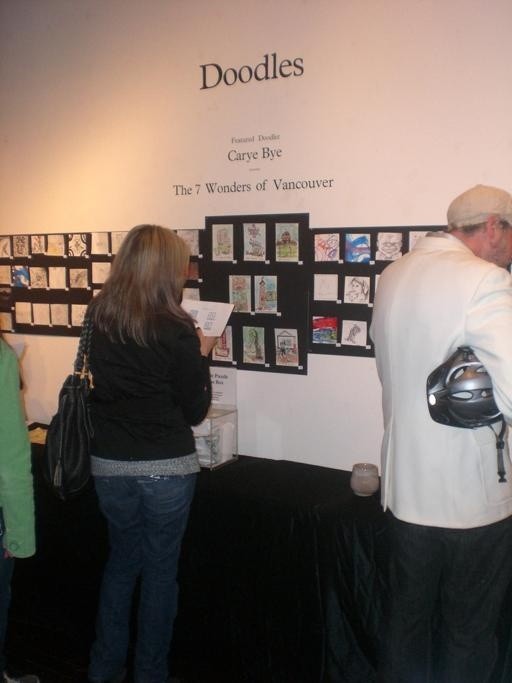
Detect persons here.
[84,224,217,683]
[368,183,512,681]
[0,325,35,629]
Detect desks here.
[27,423,385,683]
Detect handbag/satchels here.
[45,373,97,501]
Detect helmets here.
[426,347,503,427]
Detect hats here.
[446,184,512,230]
